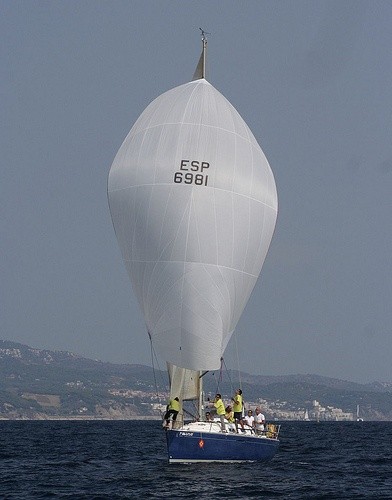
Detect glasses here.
[215,396,220,398]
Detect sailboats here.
[105,27,283,464]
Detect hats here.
[248,410,253,413]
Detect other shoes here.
[242,431,247,435]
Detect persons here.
[210,393,225,431]
[206,412,211,421]
[163,397,182,429]
[225,403,266,435]
[230,388,246,434]
